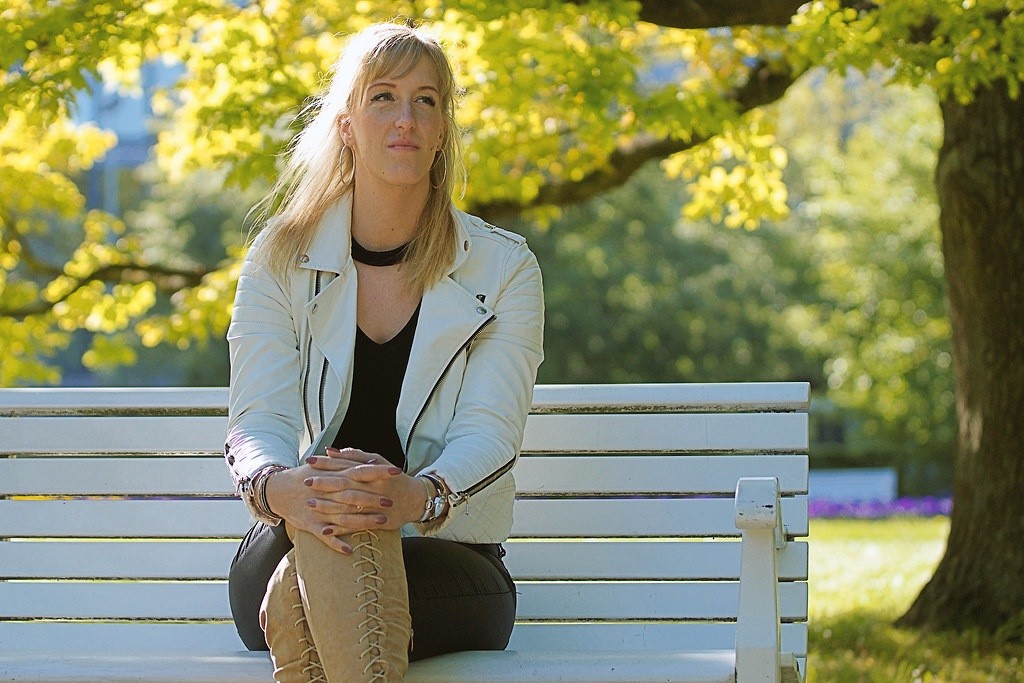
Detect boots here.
[259,546,326,680]
[283,516,412,683]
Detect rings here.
[356,505,363,512]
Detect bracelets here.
[253,467,286,520]
[419,477,433,523]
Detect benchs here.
[0,379,812,683]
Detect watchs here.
[418,473,447,523]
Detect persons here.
[223,21,545,683]
